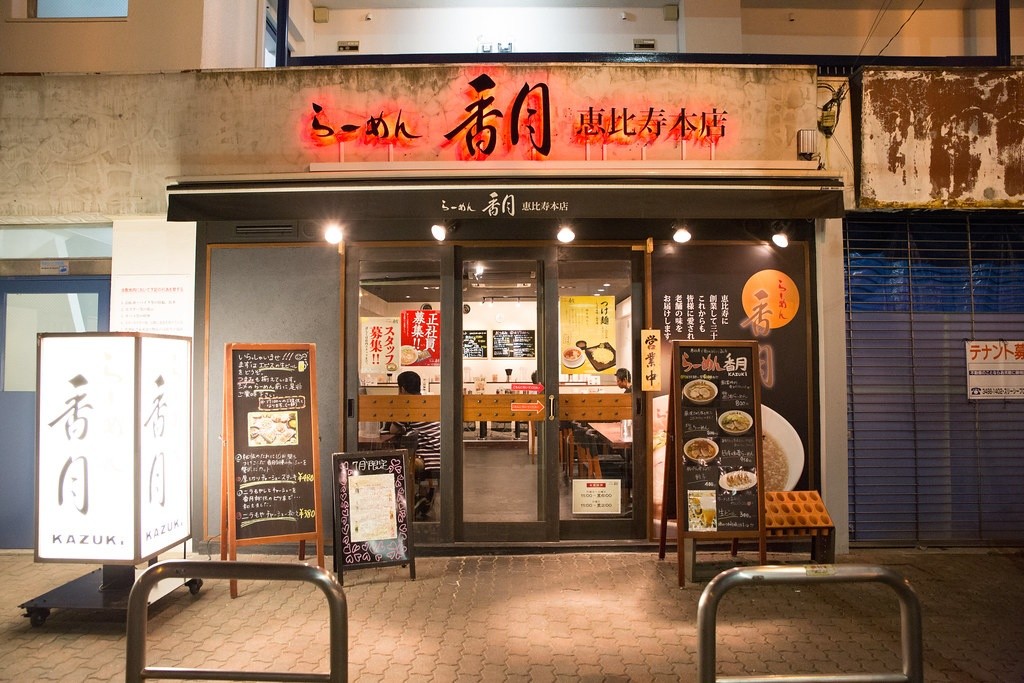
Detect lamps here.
[33,332,192,590]
[431,220,457,241]
[672,222,692,243]
[772,224,796,248]
[556,219,577,243]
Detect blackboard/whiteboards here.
[462,330,488,359]
[492,330,536,359]
[333,449,414,570]
[672,340,766,539]
[225,343,322,546]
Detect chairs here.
[531,421,626,479]
[400,431,419,521]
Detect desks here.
[587,423,632,449]
[359,433,398,449]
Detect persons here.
[614,368,632,393]
[387,370,441,519]
